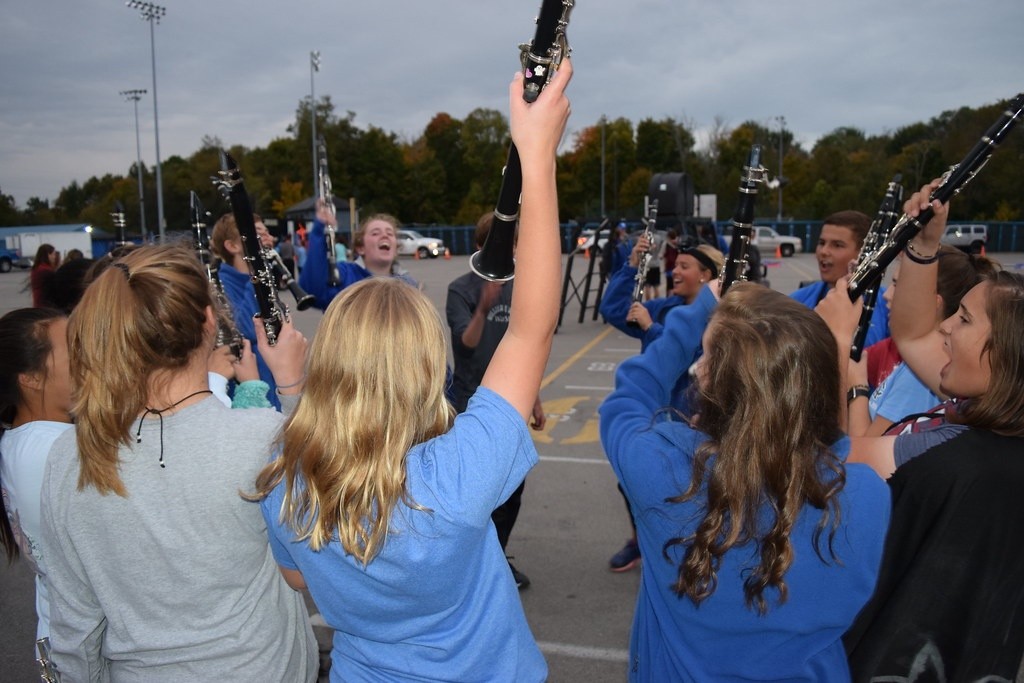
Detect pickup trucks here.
[723,226,803,259]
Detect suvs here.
[941,224,990,254]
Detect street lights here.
[118,87,151,243]
[773,114,788,222]
[309,49,323,203]
[599,114,612,221]
[980,245,986,256]
[123,1,168,245]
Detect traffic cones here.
[775,245,783,260]
[414,250,420,261]
[444,247,451,261]
[584,248,591,259]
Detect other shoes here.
[505,555,529,591]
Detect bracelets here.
[903,240,941,264]
[847,385,870,401]
[276,376,304,388]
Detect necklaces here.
[137,390,214,469]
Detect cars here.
[0,241,21,274]
[392,229,446,259]
[576,220,642,253]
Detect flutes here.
[468,0,583,282]
[626,180,666,322]
[320,137,345,282]
[850,173,906,364]
[845,93,1024,304]
[209,147,294,344]
[716,147,769,300]
[188,191,244,359]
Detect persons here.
[41,245,321,683]
[298,200,452,395]
[787,210,890,349]
[31,244,96,315]
[597,279,891,683]
[445,213,546,591]
[813,178,1024,683]
[598,238,724,571]
[212,212,282,414]
[864,338,903,388]
[86,245,271,409]
[608,228,729,300]
[237,56,575,683]
[0,309,238,683]
[276,234,347,279]
[847,243,1002,437]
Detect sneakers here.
[608,539,642,572]
